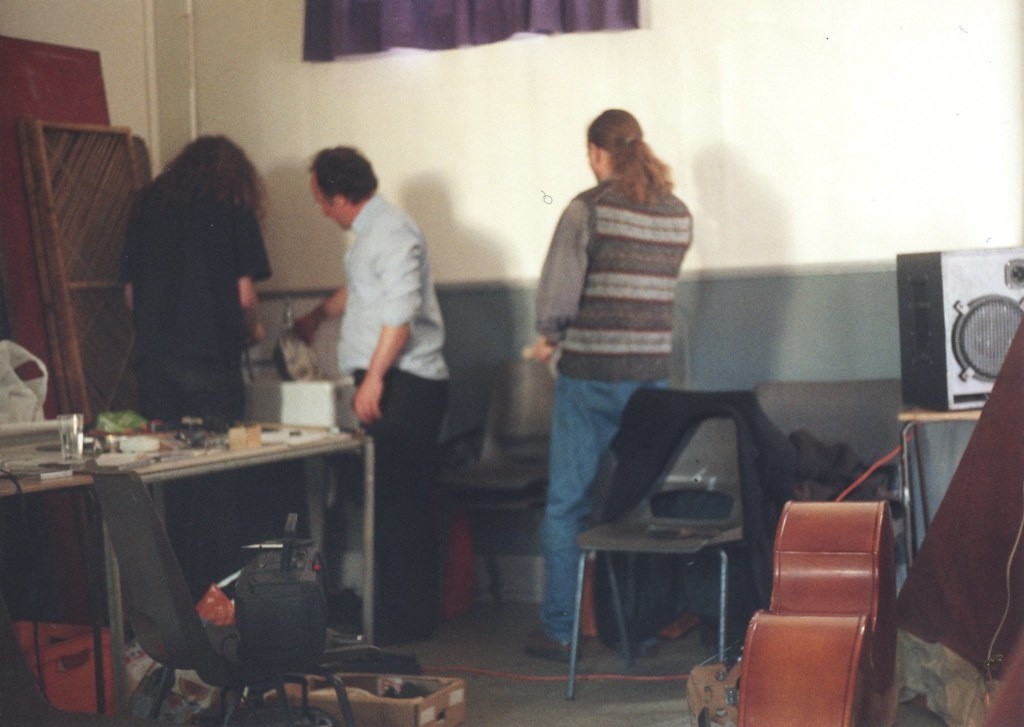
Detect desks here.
[898,407,983,576]
[0,426,375,727]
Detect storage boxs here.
[263,673,466,727]
[242,377,362,430]
[10,620,117,715]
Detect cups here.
[57,413,84,461]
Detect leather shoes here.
[522,635,584,662]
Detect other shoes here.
[358,612,443,644]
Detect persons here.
[121,134,272,602]
[293,145,450,648]
[529,108,692,661]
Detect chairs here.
[436,357,744,700]
[0,470,380,727]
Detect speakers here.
[897,248,1024,412]
